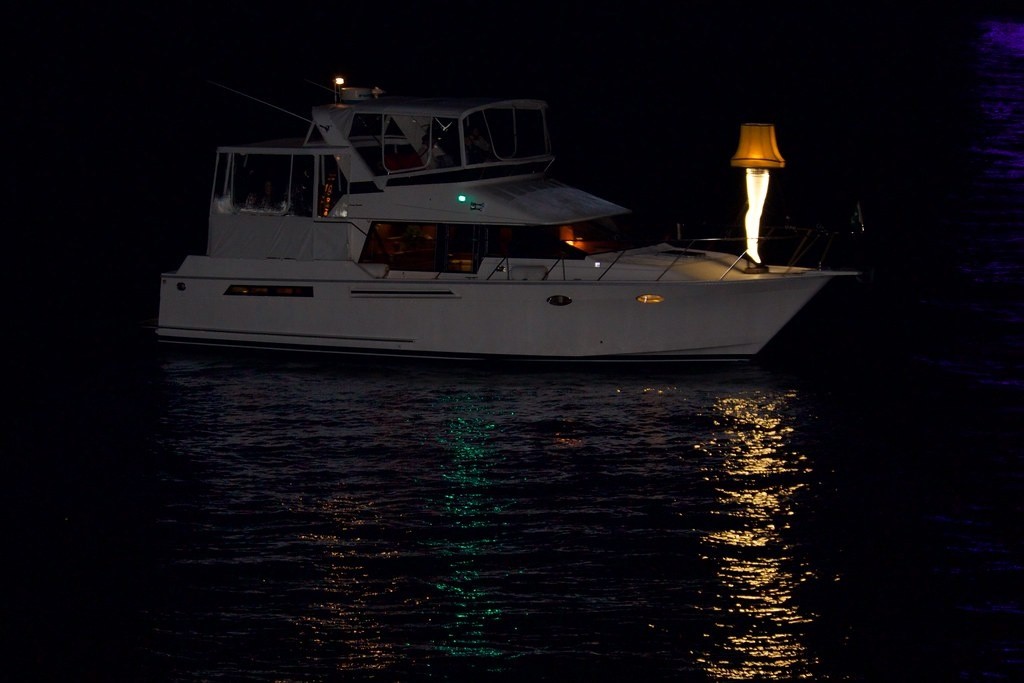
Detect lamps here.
[731,124,785,274]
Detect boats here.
[154,88,859,378]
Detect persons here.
[243,180,277,209]
[416,121,508,180]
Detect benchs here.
[382,152,426,175]
